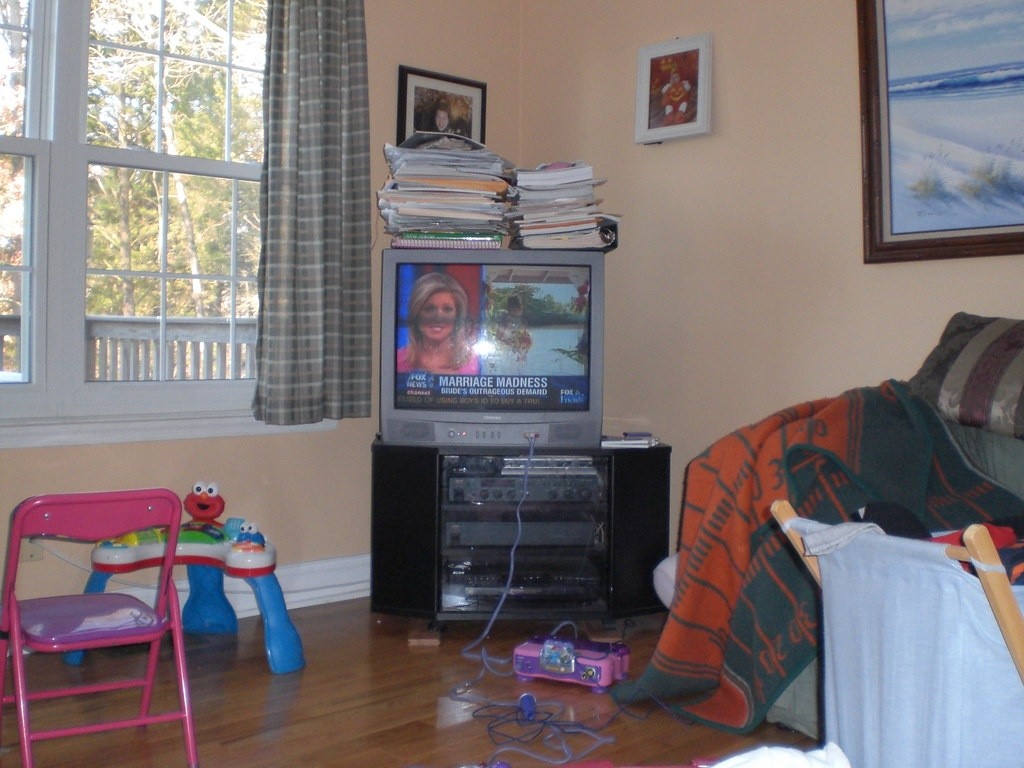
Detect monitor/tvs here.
[379,248,605,450]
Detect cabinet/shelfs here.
[368,444,673,632]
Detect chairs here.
[1,480,202,767]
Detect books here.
[377,130,617,253]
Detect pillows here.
[910,309,1024,442]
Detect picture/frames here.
[856,1,1024,264]
[395,63,488,150]
[635,31,712,143]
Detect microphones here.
[520,694,537,721]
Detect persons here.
[395,272,480,374]
[662,72,690,124]
[496,294,530,361]
[425,97,455,134]
